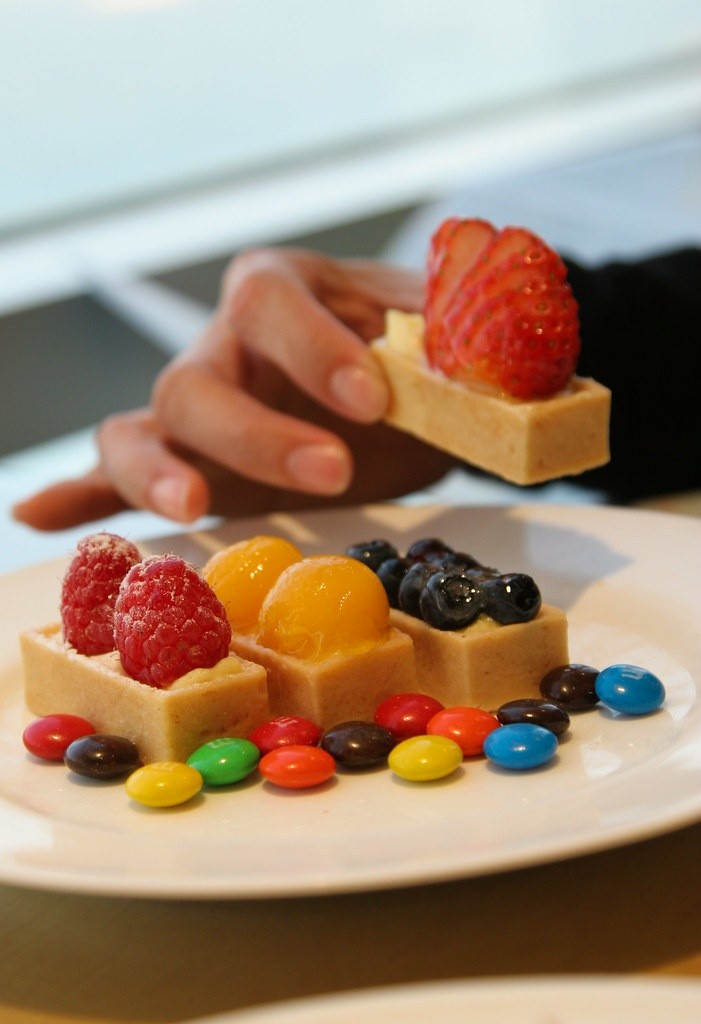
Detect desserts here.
[356,214,610,486]
[20,531,569,768]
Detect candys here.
[20,663,665,809]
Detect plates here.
[179,972,701,1024]
[0,504,701,899]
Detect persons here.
[8,243,701,532]
